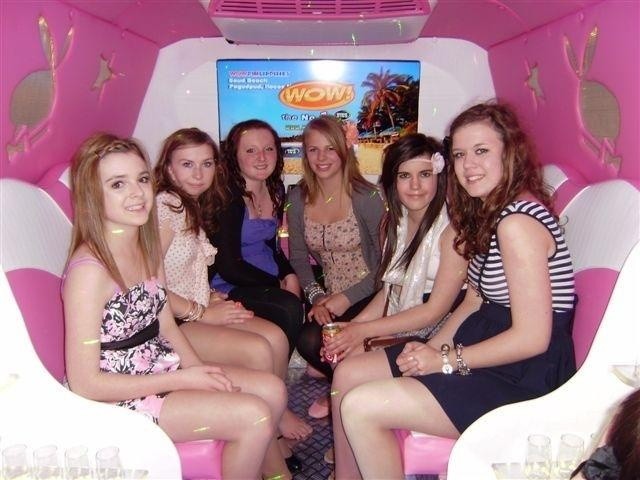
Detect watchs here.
[440,342,453,375]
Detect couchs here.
[1,157,257,479]
[374,160,640,474]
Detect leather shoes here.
[304,362,334,419]
[273,433,303,474]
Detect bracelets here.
[175,299,206,323]
[303,280,325,305]
[455,343,471,376]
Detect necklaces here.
[256,205,262,217]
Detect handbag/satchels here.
[361,283,426,348]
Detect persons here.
[331,102,579,480]
[285,114,387,420]
[207,118,313,441]
[319,133,455,480]
[154,127,313,480]
[59,130,288,480]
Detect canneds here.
[321,325,343,364]
